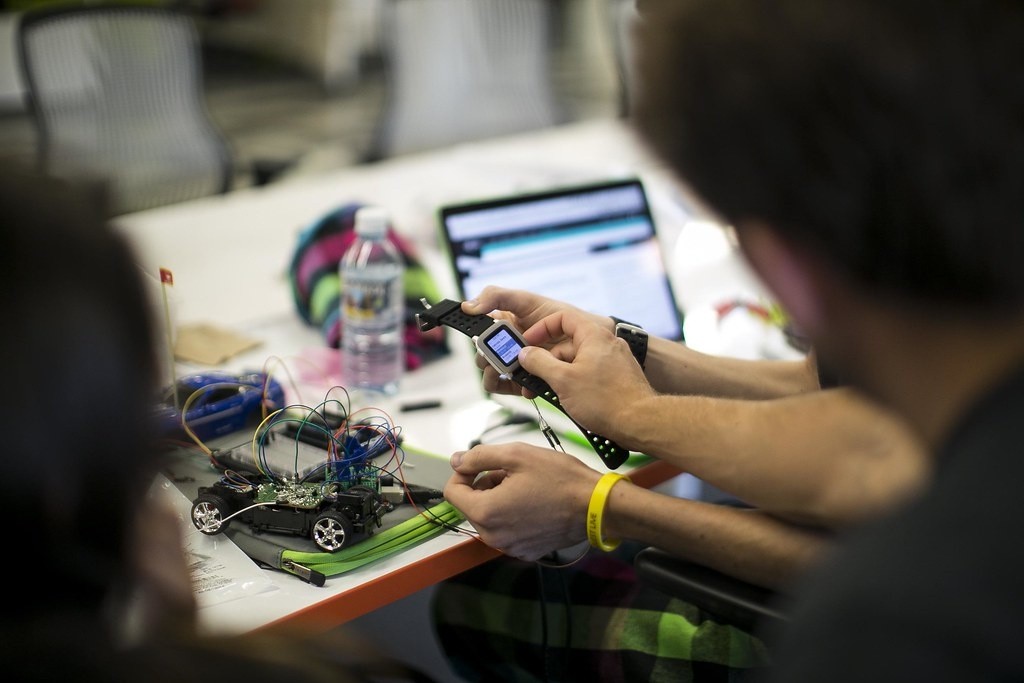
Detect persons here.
[444,0,1024,683]
[2,139,439,683]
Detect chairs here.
[14,0,643,218]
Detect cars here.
[155,372,285,449]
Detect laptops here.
[435,175,692,467]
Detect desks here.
[108,115,805,644]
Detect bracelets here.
[584,470,632,555]
[604,306,650,382]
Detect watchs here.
[402,290,630,470]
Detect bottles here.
[340,206,404,406]
